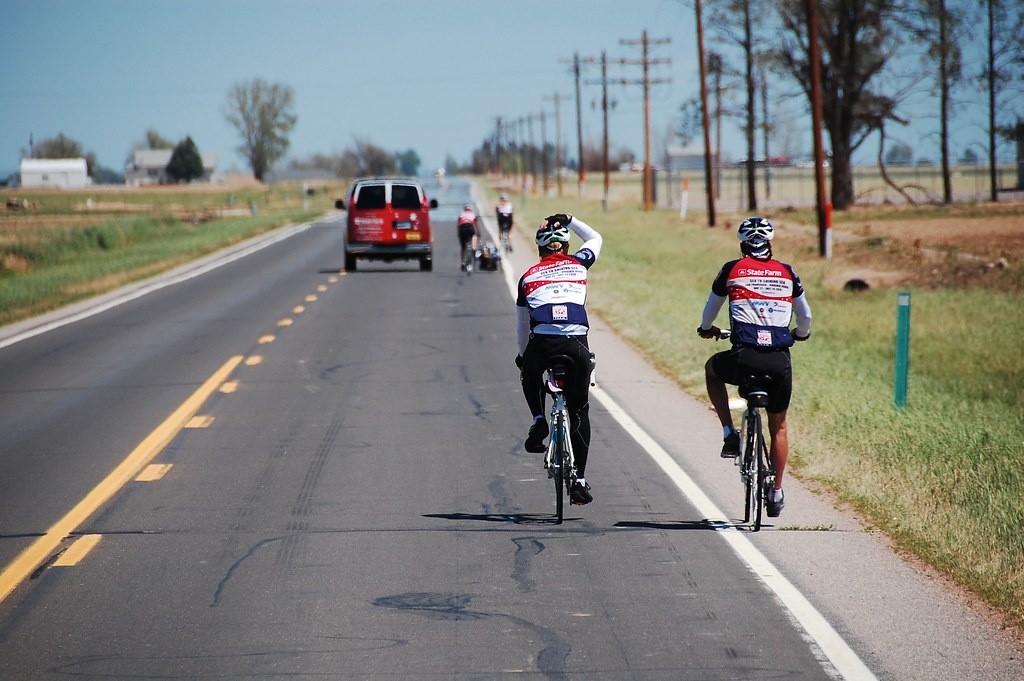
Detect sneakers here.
[721,433,741,457]
[570,481,593,505]
[766,488,784,517]
[524,417,550,452]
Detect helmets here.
[536,221,571,248]
[463,202,471,210]
[498,193,508,200]
[737,216,775,248]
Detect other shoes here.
[461,262,466,272]
[508,245,514,253]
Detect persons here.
[456,204,481,274]
[516,214,603,506]
[699,216,812,518]
[495,194,514,252]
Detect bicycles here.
[463,233,480,276]
[515,331,596,524]
[498,219,513,253]
[698,323,809,533]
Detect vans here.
[335,176,439,272]
